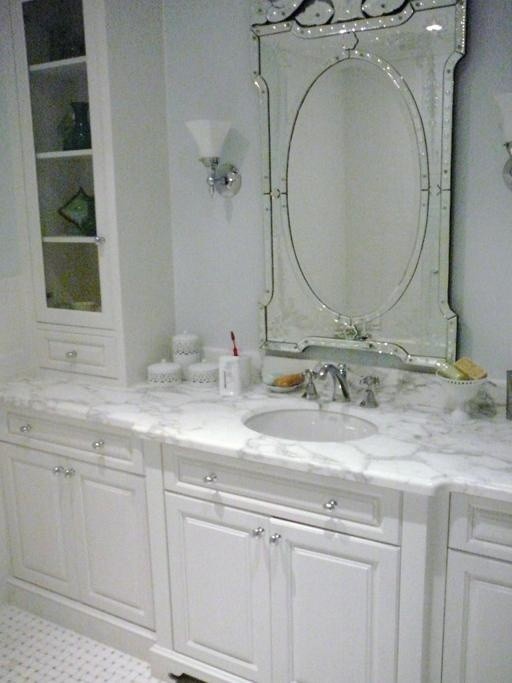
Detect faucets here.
[317,362,353,403]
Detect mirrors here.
[248,0,468,372]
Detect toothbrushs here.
[231,331,238,356]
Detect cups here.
[218,354,252,397]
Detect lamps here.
[489,91,512,189]
[183,115,241,199]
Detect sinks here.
[240,402,378,444]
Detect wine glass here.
[435,367,488,424]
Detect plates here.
[266,381,308,391]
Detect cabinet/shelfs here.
[10,1,177,384]
[439,491,511,683]
[164,449,426,682]
[0,411,151,628]
